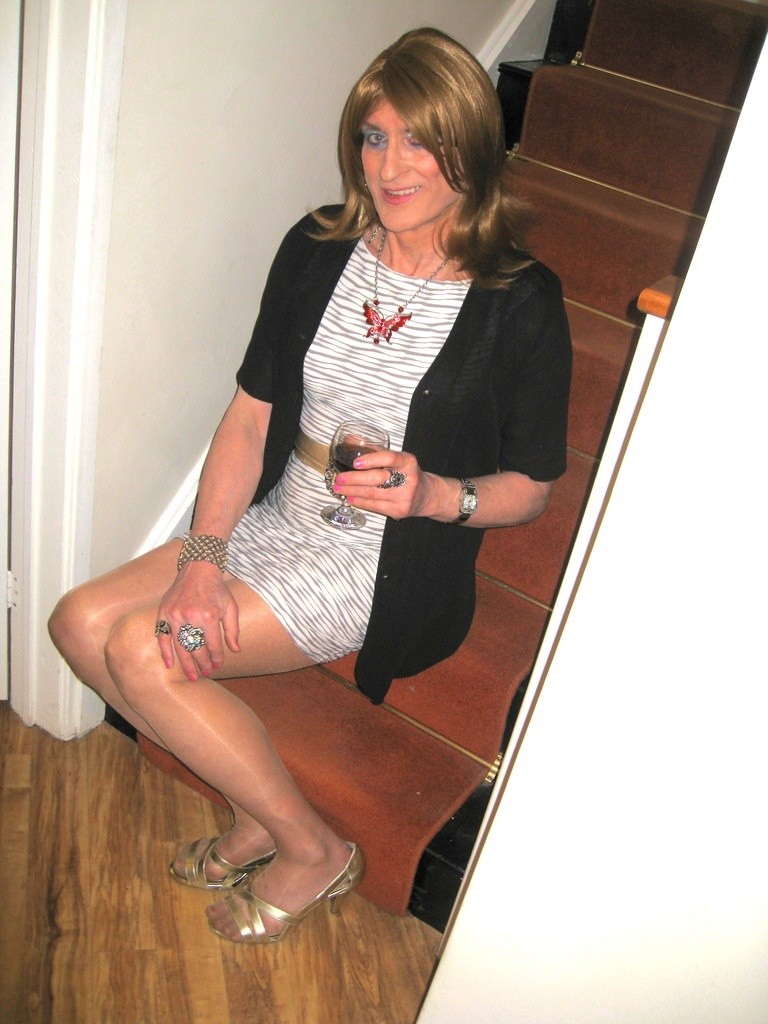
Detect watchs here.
[441,476,478,525]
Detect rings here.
[379,468,407,489]
[155,621,172,637]
[177,624,206,651]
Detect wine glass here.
[322,421,390,529]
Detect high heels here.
[168,833,278,892]
[207,841,366,944]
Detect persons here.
[48,29,575,944]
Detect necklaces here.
[362,230,447,345]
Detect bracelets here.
[178,534,229,575]
